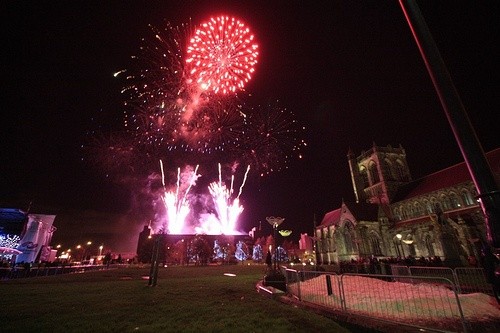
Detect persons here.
[335,254,447,275]
[266,249,272,273]
[2,256,92,274]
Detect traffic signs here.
[147,238,160,287]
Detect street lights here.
[265,217,284,276]
[279,230,291,266]
[396,233,406,260]
